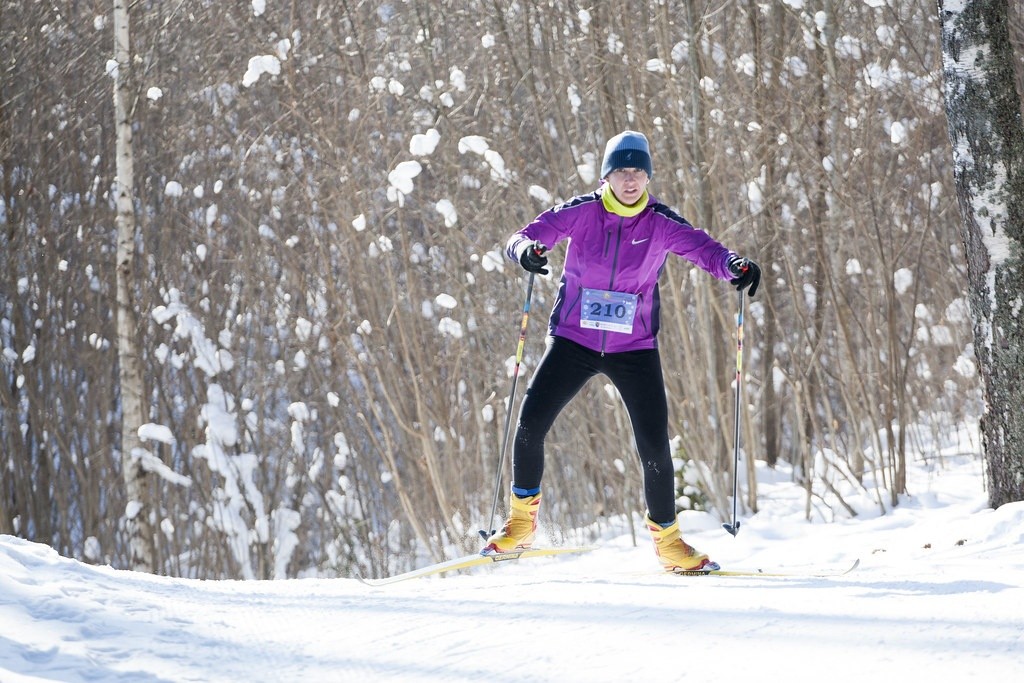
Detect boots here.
[646,509,709,573]
[488,490,542,555]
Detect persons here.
[486,130,762,570]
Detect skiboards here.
[478,528,721,579]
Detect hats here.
[601,130,653,180]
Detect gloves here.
[728,258,760,297]
[521,244,549,275]
